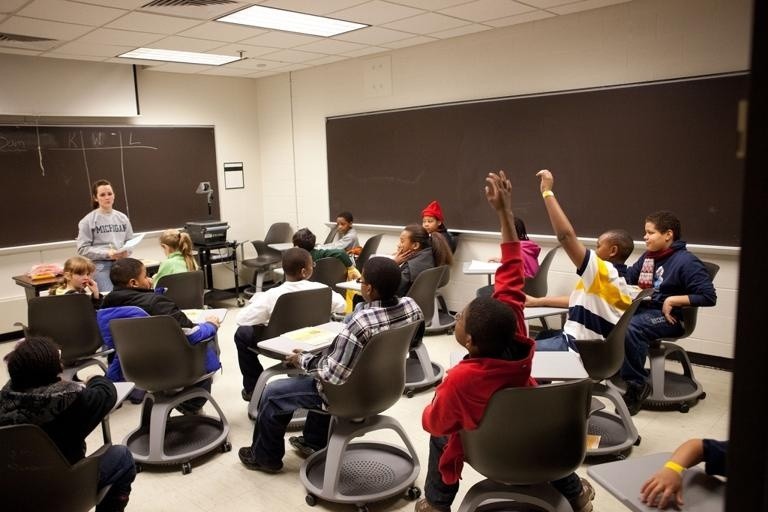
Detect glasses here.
[356,278,364,283]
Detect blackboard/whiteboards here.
[0,123,224,255]
[323,70,747,256]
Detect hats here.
[422,200,443,221]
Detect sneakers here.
[175,405,203,416]
[570,478,595,512]
[242,389,253,400]
[239,447,283,473]
[415,498,450,512]
[289,436,315,455]
[616,383,650,416]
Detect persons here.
[232,201,453,472]
[0,336,136,512]
[147,228,200,297]
[638,437,731,511]
[76,179,137,292]
[94,257,222,419]
[46,256,103,326]
[413,171,716,512]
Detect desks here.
[586,450,726,510]
